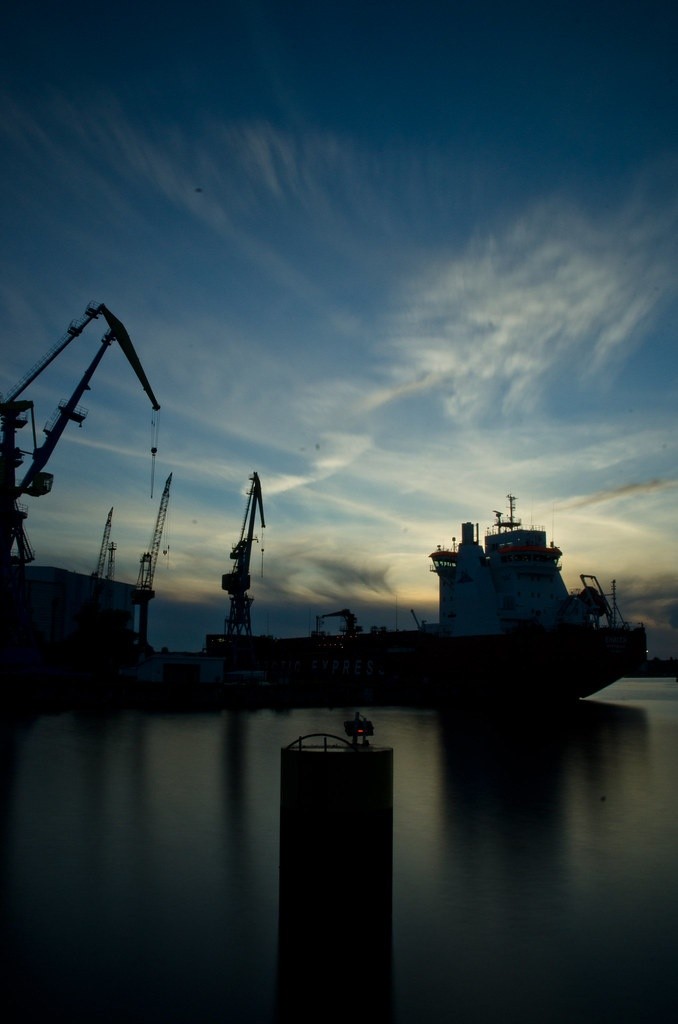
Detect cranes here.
[226,472,272,636]
[0,290,163,575]
[105,540,121,579]
[133,473,174,664]
[86,503,113,577]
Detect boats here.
[166,493,649,713]
